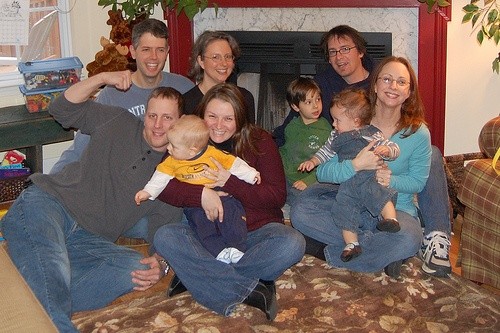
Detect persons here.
[272,25,454,279]
[0,70,184,333]
[150,84,306,322]
[183,30,256,126]
[298,86,401,262]
[48,18,196,241]
[291,56,431,278]
[135,115,262,264]
[278,77,336,205]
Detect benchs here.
[0,104,74,174]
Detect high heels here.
[244,280,275,322]
[168,272,187,297]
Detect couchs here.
[456,114,500,289]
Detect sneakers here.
[420,234,452,278]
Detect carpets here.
[71,254,500,333]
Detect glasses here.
[376,76,411,86]
[327,46,358,57]
[203,55,235,62]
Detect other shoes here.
[376,219,400,233]
[384,260,402,279]
[341,243,362,262]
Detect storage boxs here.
[18,56,84,89]
[19,85,66,114]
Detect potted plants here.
[99,0,218,57]
